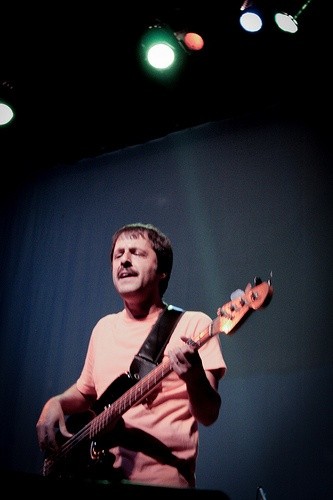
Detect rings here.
[175,358,179,363]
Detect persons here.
[35,222,227,488]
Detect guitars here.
[41,276,273,494]
